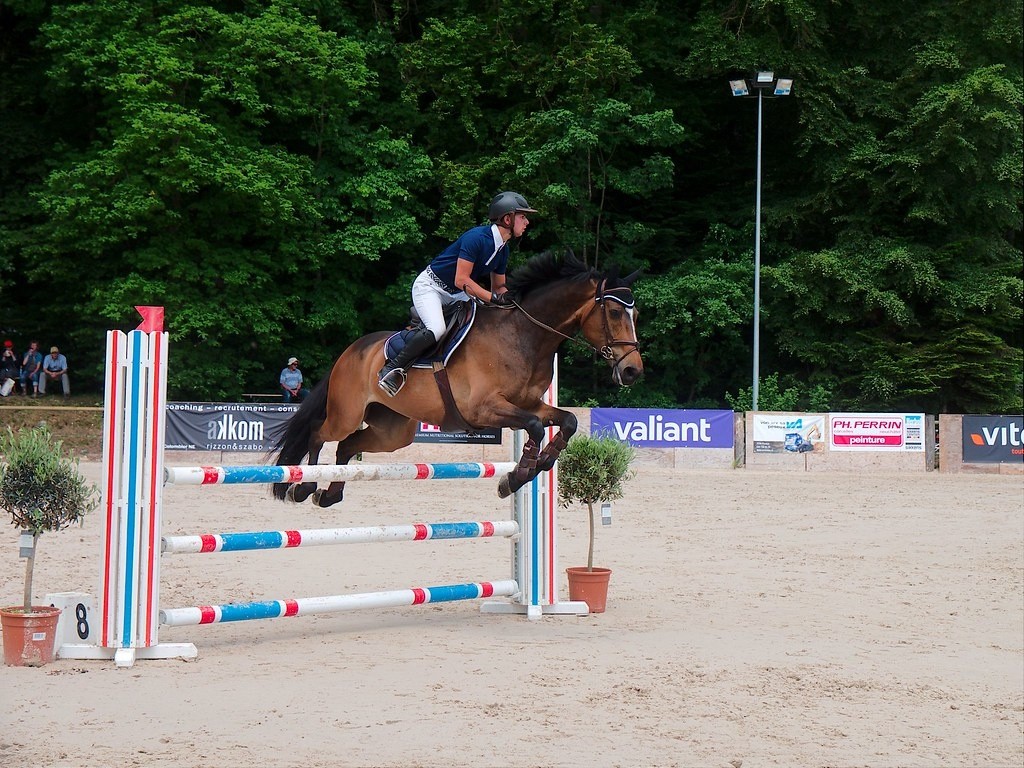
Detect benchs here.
[241,393,284,402]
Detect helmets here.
[489,192,538,228]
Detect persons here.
[19,342,42,398]
[376,191,538,392]
[280,358,310,403]
[0,338,18,396]
[38,347,71,396]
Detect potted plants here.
[0,424,102,667]
[558,420,637,614]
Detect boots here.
[376,328,436,393]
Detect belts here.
[426,265,454,294]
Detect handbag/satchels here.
[6,367,18,377]
[0,378,15,396]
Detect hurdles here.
[57,328,590,661]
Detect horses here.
[257,249,646,508]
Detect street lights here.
[730,68,795,411]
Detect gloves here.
[491,289,520,305]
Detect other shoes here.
[39,392,43,397]
[21,391,27,397]
[10,391,16,396]
[31,393,37,398]
[64,394,70,400]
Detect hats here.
[50,347,58,354]
[3,340,13,350]
[287,357,299,366]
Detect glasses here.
[293,364,298,366]
